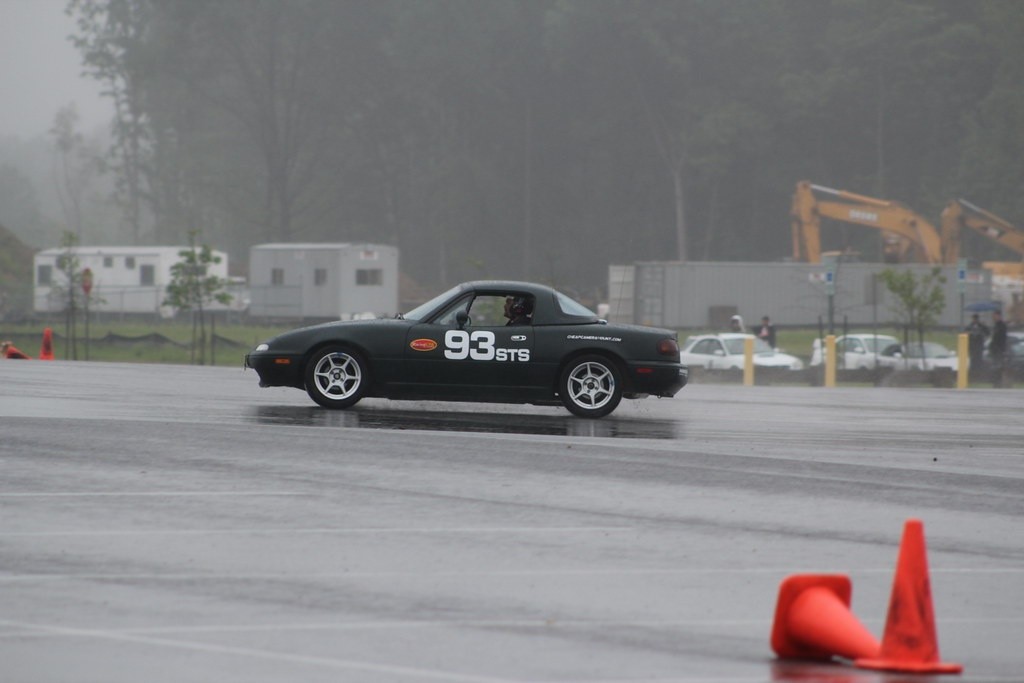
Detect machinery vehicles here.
[783,178,1022,277]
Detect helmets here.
[505,294,533,314]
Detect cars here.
[982,331,1024,367]
[809,333,906,380]
[242,279,688,421]
[873,340,962,388]
[679,331,805,382]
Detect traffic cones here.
[38,327,54,361]
[769,572,881,657]
[6,345,32,361]
[854,517,964,675]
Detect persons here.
[503,294,533,327]
[729,314,747,334]
[989,314,1007,387]
[964,315,989,385]
[757,315,777,350]
